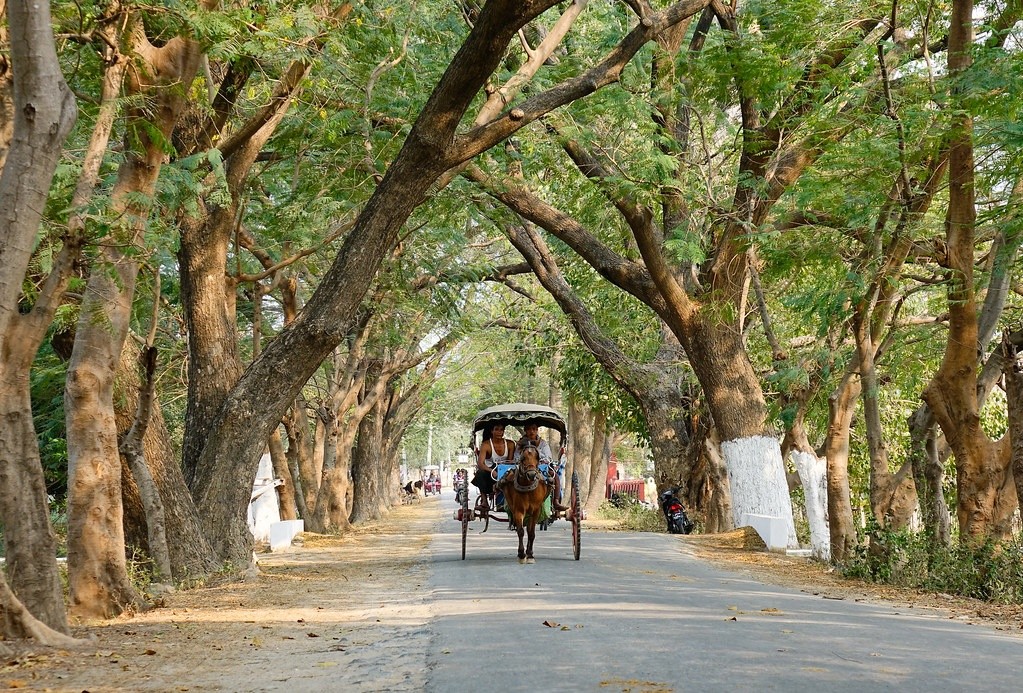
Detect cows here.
[403,480,423,505]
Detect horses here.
[503,437,548,564]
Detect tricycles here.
[421,464,442,497]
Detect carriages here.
[453,401,588,561]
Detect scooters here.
[454,480,469,506]
[656,484,694,535]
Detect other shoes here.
[475,504,490,512]
[553,505,569,511]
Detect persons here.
[405,466,468,496]
[512,422,569,511]
[471,421,516,510]
